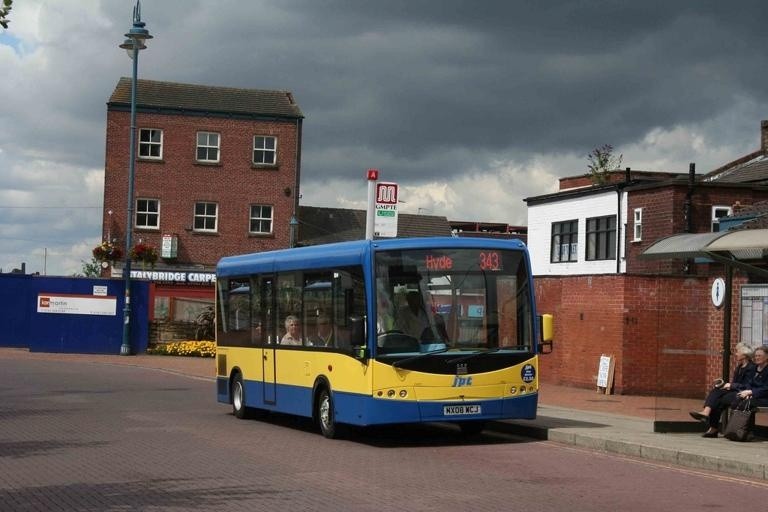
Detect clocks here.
[711,278,726,308]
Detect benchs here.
[722,407,767,437]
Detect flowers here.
[129,244,158,264]
[91,241,118,261]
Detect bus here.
[213,237,555,440]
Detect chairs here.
[348,317,367,347]
[235,307,250,330]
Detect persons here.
[689,341,757,438]
[374,298,396,334]
[279,313,313,345]
[730,346,767,442]
[305,313,344,349]
[394,290,454,345]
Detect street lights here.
[119,0,153,356]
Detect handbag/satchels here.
[723,397,752,441]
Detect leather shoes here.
[688,412,709,422]
[702,431,719,438]
